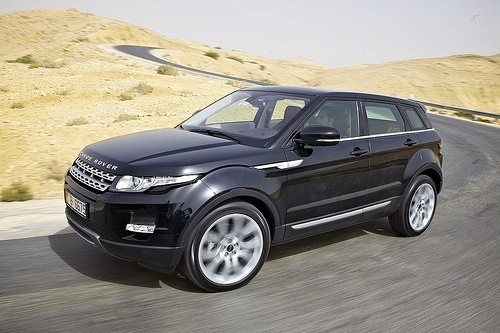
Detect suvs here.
[61,85,445,293]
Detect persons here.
[317,106,338,128]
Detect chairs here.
[270,106,302,132]
